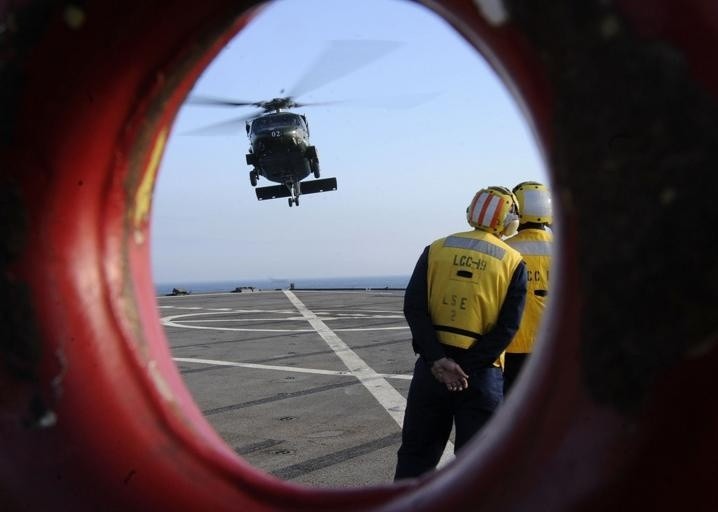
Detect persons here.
[502,180,556,400]
[388,183,527,482]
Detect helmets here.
[513,181,552,225]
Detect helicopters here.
[177,39,442,207]
